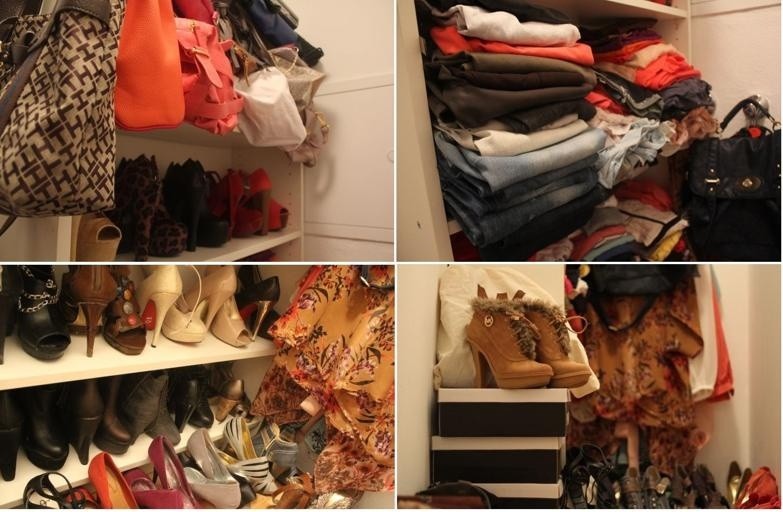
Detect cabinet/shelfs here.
[396,0,695,260]
[2,119,306,262]
[0,264,309,509]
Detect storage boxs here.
[431,435,568,498]
[436,385,573,437]
[467,478,562,510]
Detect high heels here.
[466,284,590,390]
[78,154,287,261]
[0,265,315,508]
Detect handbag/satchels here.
[0,0,329,217]
[686,97,781,262]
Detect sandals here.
[562,444,751,509]
